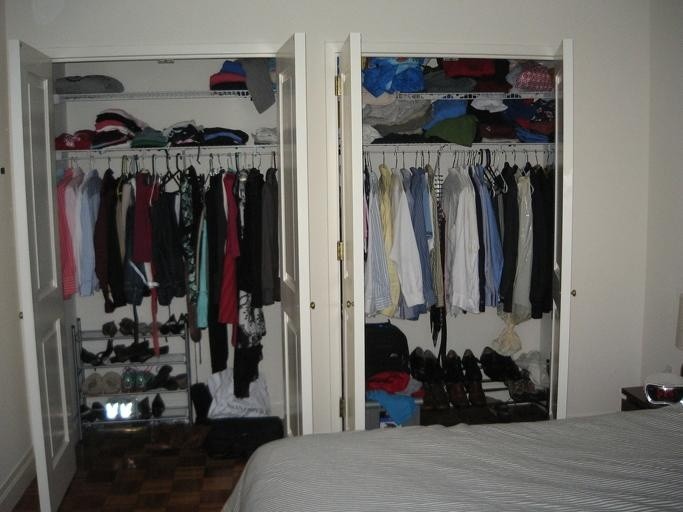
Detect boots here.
[171,383,212,450]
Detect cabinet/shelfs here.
[6,32,312,512]
[324,30,572,431]
[70,315,193,430]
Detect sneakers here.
[79,312,186,422]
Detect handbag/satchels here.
[207,417,284,459]
[366,324,409,371]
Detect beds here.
[222,401,683,512]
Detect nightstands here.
[620,386,683,412]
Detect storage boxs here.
[365,398,423,431]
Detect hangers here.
[365,143,553,171]
[67,144,278,192]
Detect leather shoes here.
[411,347,550,411]
